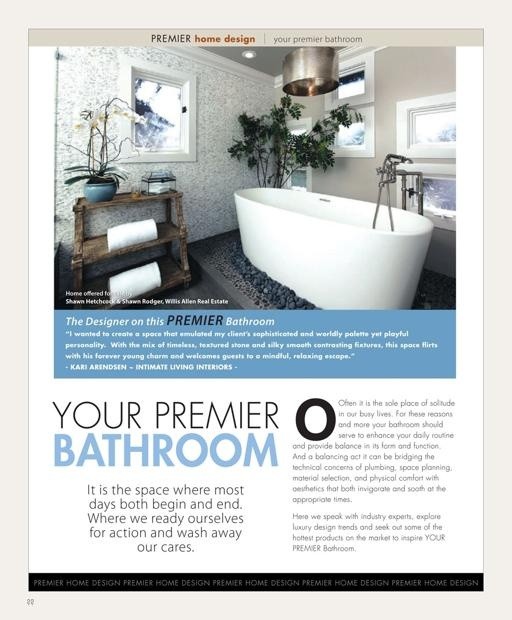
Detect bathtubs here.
[231,187,435,310]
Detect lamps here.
[281,48,341,98]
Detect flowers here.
[63,96,148,188]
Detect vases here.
[83,183,116,203]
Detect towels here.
[108,261,162,307]
[106,218,159,252]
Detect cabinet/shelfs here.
[70,191,192,310]
